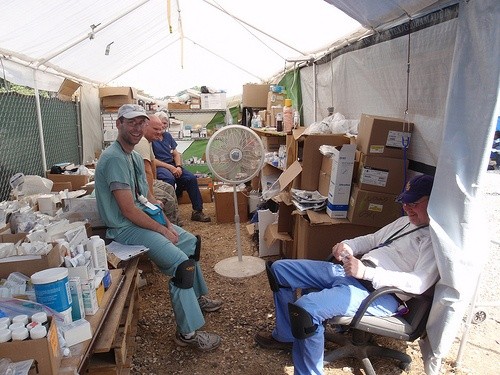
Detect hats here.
[394,174,434,204]
[117,103,150,121]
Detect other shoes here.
[191,210,211,222]
[254,331,293,349]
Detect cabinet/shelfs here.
[101,109,229,152]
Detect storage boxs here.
[99,86,133,108]
[0,161,98,375]
[179,83,414,265]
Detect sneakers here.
[198,295,224,312]
[174,330,220,352]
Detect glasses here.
[400,198,428,208]
[127,119,148,128]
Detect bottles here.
[251,98,300,133]
[0,195,110,360]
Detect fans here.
[206,125,266,278]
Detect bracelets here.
[175,165,183,168]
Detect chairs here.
[322,247,434,375]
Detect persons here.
[255,172,440,375]
[133,115,186,229]
[92,102,224,354]
[154,111,213,222]
